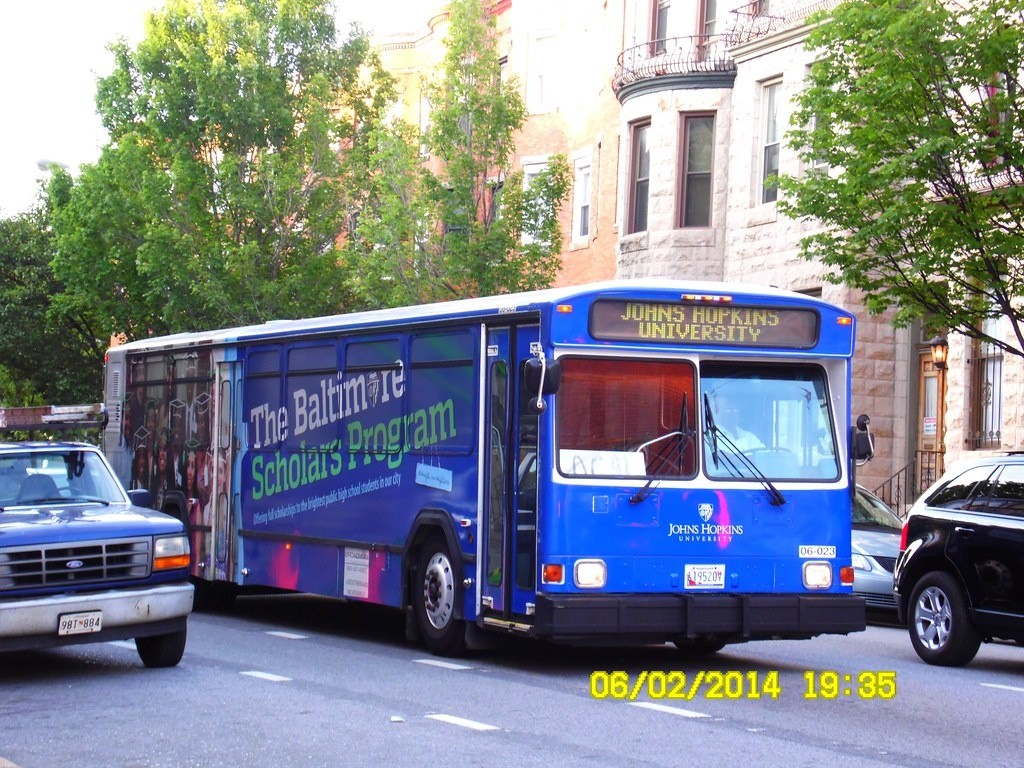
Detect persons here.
[122,350,230,580]
[714,401,769,458]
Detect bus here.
[103,281,875,662]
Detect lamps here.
[929,335,949,370]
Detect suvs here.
[1,402,197,669]
[892,450,1024,667]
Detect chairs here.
[17,473,61,500]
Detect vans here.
[851,482,904,625]
